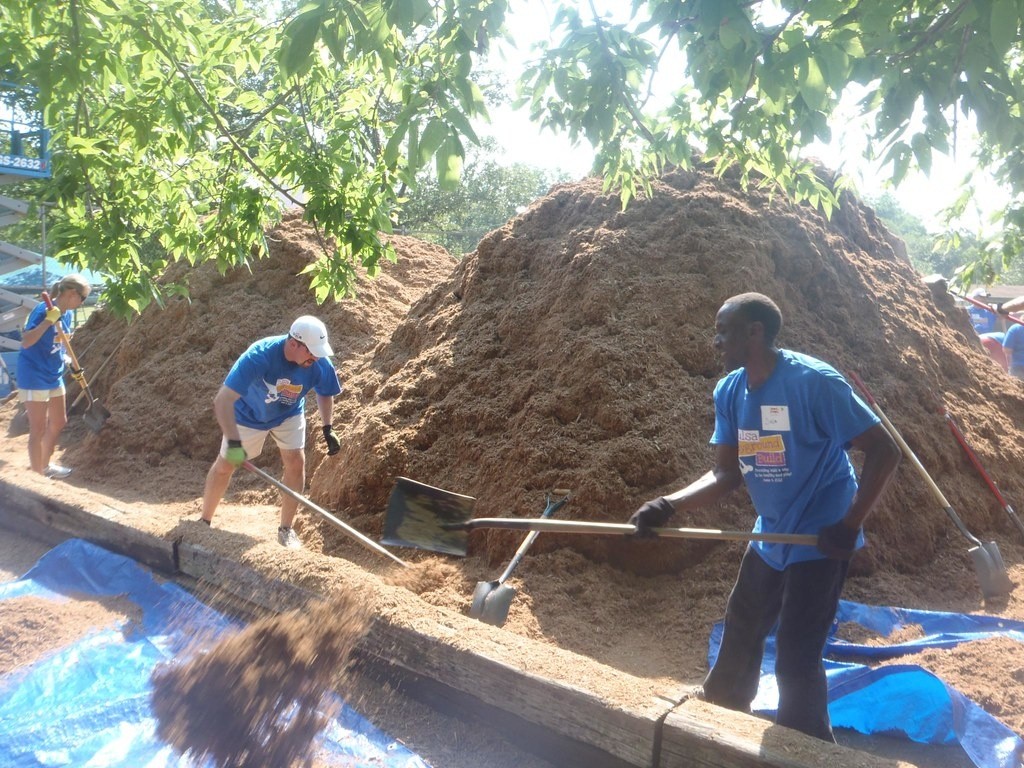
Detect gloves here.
[67,358,85,382]
[817,519,861,561]
[624,496,675,544]
[226,440,247,468]
[322,425,340,456]
[45,306,60,325]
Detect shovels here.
[947,289,1024,326]
[848,369,1015,602]
[42,292,112,432]
[243,459,416,569]
[378,477,817,560]
[468,489,573,630]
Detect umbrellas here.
[0,257,134,289]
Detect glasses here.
[69,288,86,302]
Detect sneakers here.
[278,528,301,549]
[43,464,71,478]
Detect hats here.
[973,287,991,299]
[290,315,334,357]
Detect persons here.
[195,315,341,550]
[16,276,90,481]
[624,292,902,744]
[967,287,1024,380]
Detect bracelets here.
[997,303,1009,315]
